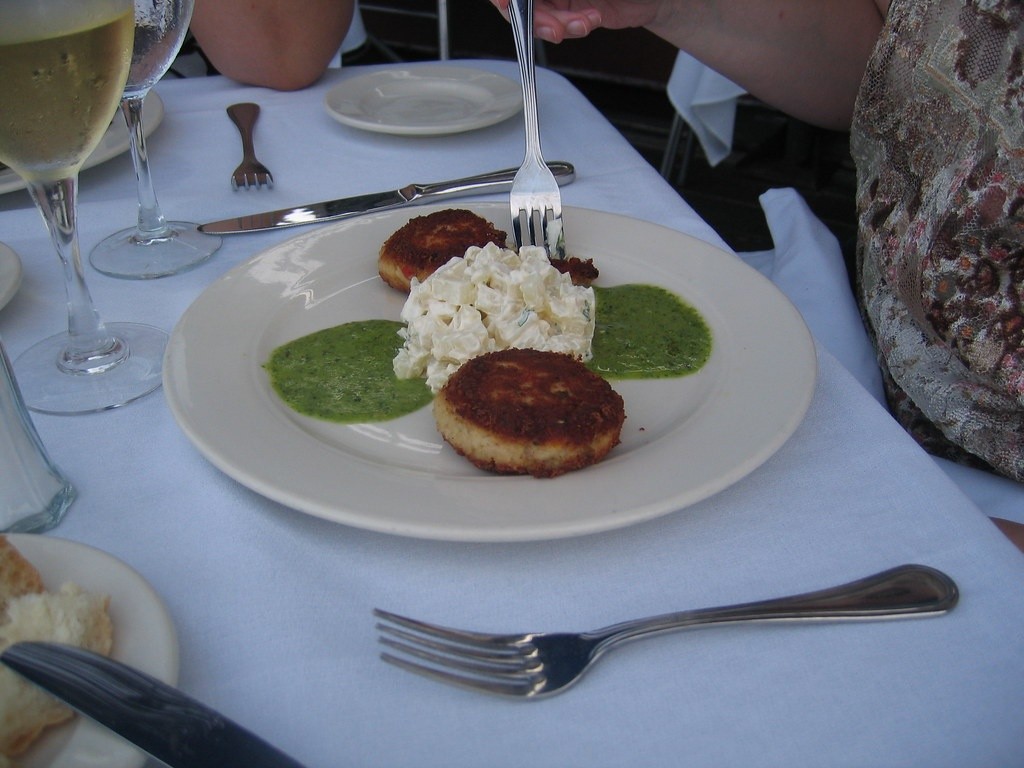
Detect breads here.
[0,537,114,757]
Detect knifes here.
[197,159,576,235]
[2,637,306,768]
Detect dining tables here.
[0,60,1024,768]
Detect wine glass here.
[87,0,225,281]
[1,0,180,417]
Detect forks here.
[373,564,959,698]
[504,0,566,263]
[222,100,276,194]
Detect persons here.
[487,0,1024,489]
[161,0,365,91]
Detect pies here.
[378,208,508,291]
[549,257,600,288]
[435,350,626,475]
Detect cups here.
[0,342,79,535]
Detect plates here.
[0,238,22,318]
[164,193,819,542]
[1,530,186,768]
[325,66,525,136]
[1,89,166,199]
